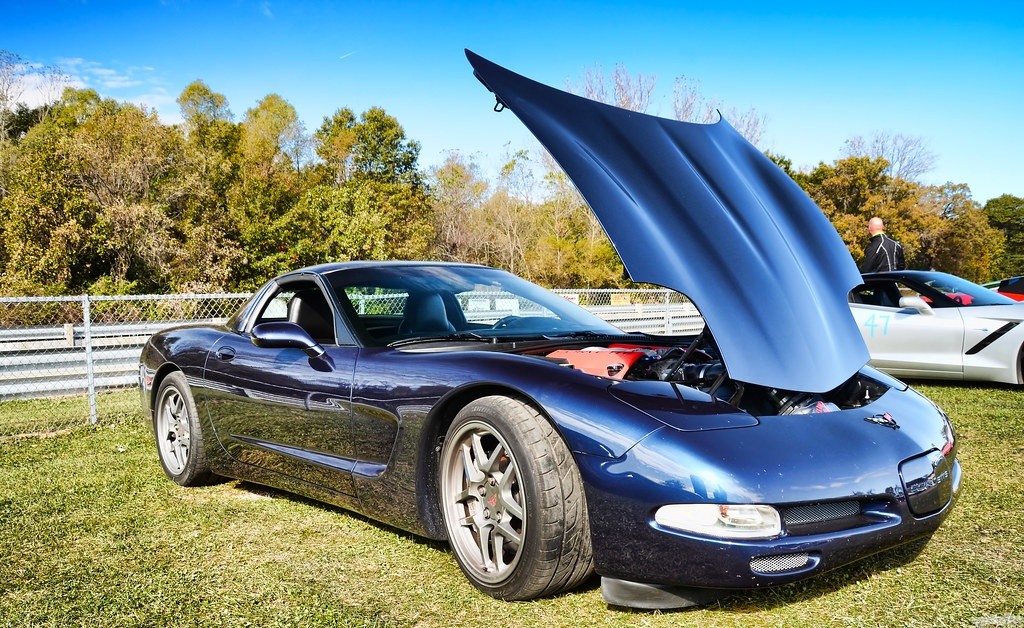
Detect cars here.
[847,270,1024,384]
[918,276,1024,306]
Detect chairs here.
[873,280,902,307]
[396,290,456,339]
[285,287,373,347]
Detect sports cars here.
[137,48,962,607]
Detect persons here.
[853,216,906,308]
